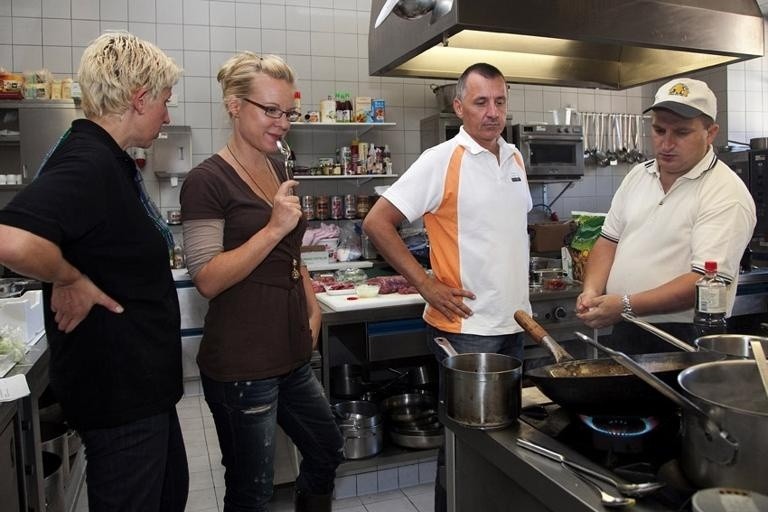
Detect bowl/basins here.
[716,144,732,154]
[691,486,768,512]
[352,282,382,298]
[532,268,567,286]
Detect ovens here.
[510,122,587,179]
[417,113,515,155]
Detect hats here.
[641,77,718,124]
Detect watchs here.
[621,292,637,320]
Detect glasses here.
[243,96,301,122]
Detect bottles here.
[693,260,730,344]
[361,229,377,261]
[289,137,393,178]
[294,91,353,124]
[172,241,184,269]
[51,78,73,101]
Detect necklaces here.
[225,140,301,282]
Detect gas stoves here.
[515,399,695,511]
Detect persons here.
[177,48,350,510]
[362,61,536,512]
[572,75,760,358]
[0,27,194,510]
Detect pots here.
[622,313,768,362]
[514,313,727,419]
[40,390,82,512]
[427,82,456,113]
[434,333,525,432]
[676,360,767,492]
[726,137,768,152]
[326,361,446,459]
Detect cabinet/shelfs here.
[289,121,399,225]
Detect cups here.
[315,238,341,264]
[21,82,48,101]
[166,211,183,224]
[0,174,23,187]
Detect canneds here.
[331,196,343,219]
[332,164,342,174]
[303,196,315,220]
[322,164,332,174]
[344,194,356,219]
[316,196,328,219]
[357,195,369,219]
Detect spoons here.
[275,138,292,180]
[560,463,636,511]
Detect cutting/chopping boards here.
[314,289,427,313]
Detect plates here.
[324,286,357,297]
